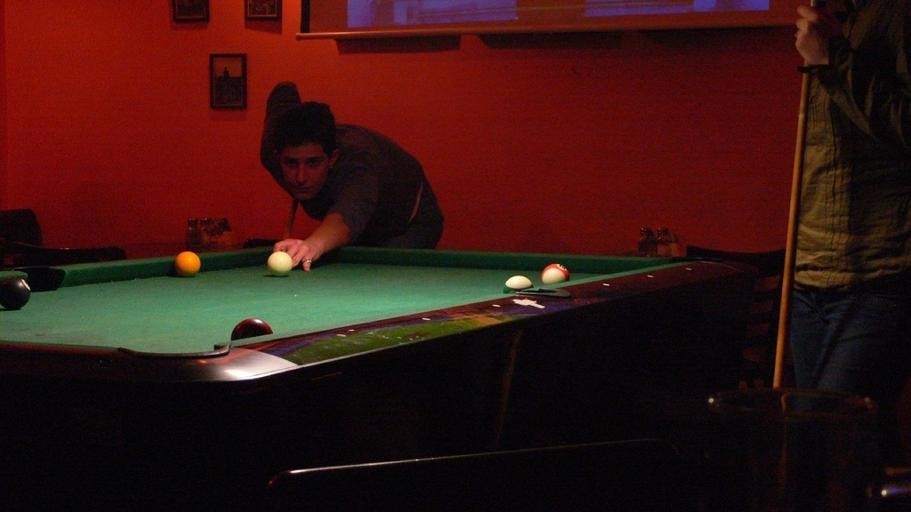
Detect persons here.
[258,74,446,274]
[777,2,911,422]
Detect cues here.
[773,63,811,387]
[280,200,298,239]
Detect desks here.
[0,240,748,511]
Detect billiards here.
[1,278,30,309]
[541,264,569,284]
[503,275,533,295]
[268,251,296,275]
[231,319,273,338]
[176,251,200,277]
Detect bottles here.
[186,215,217,249]
[637,225,674,258]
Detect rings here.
[300,258,312,266]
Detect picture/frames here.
[175,0,210,23]
[243,0,281,20]
[208,52,249,110]
